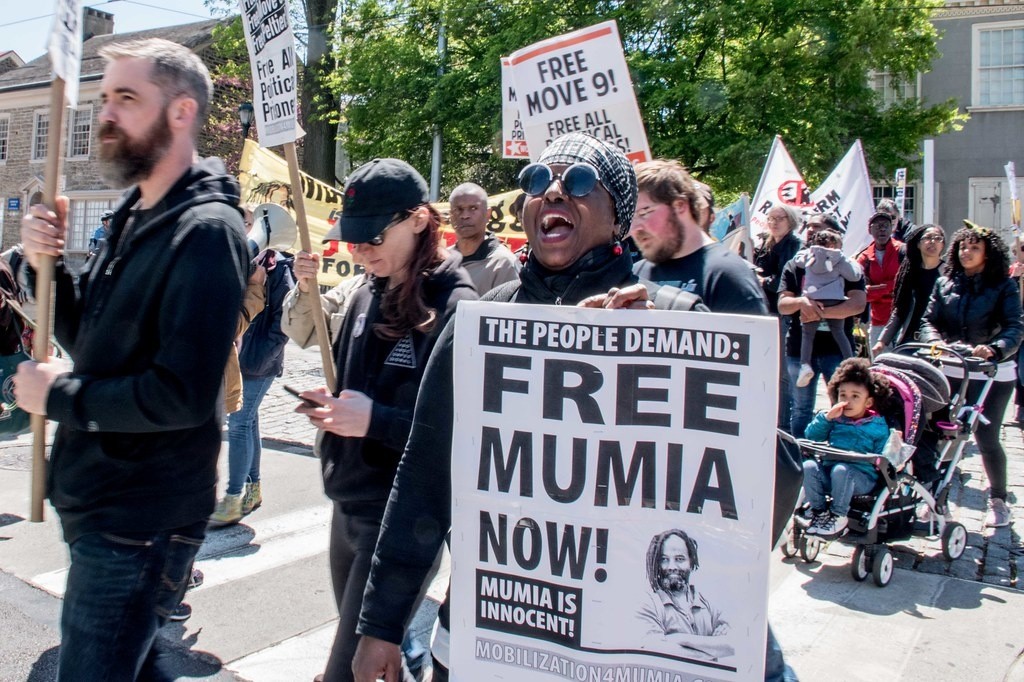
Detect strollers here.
[777,338,1000,588]
[0,259,66,408]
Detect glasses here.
[871,222,891,229]
[767,216,788,222]
[919,235,945,243]
[370,208,417,246]
[516,162,612,198]
[890,214,896,220]
[244,222,252,227]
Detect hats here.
[321,159,430,244]
[691,180,715,209]
[536,133,638,243]
[868,213,892,226]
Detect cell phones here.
[283,386,320,410]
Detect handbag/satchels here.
[651,286,804,553]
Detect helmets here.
[100,210,117,221]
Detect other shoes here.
[1009,407,1024,424]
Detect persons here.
[291,156,485,682]
[11,36,252,682]
[630,528,739,666]
[0,176,1024,623]
[349,129,719,682]
[625,157,799,682]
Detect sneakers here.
[188,567,204,589]
[984,498,1013,527]
[241,481,262,516]
[918,501,952,523]
[207,493,242,526]
[796,366,814,387]
[168,602,192,621]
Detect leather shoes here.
[806,510,830,534]
[817,516,849,536]
